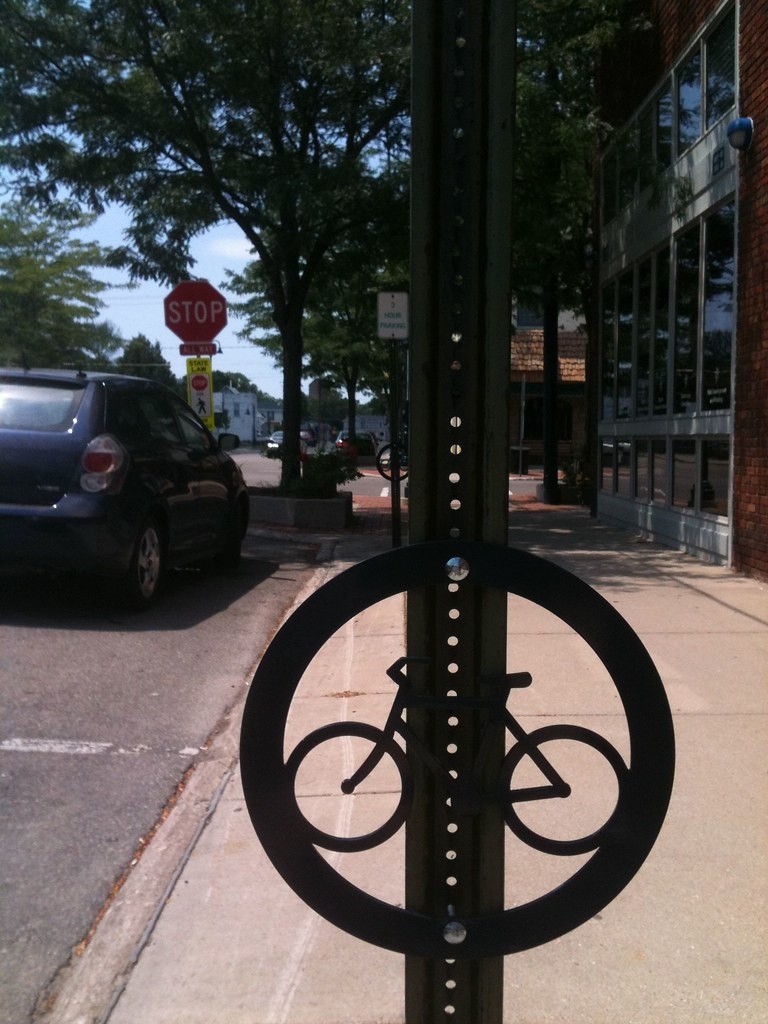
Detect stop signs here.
[162,279,228,344]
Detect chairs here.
[120,397,150,437]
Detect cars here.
[299,429,316,447]
[335,430,379,457]
[265,431,308,456]
[0,364,252,615]
